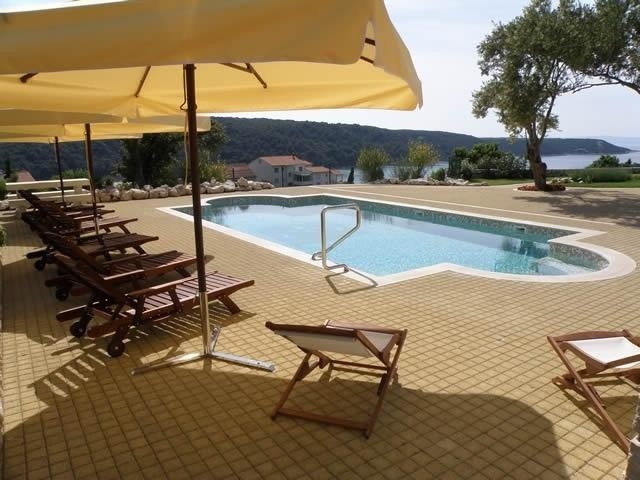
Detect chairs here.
[546,325,640,455]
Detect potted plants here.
[0,175,11,210]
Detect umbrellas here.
[1,134,147,197]
[0,0,425,292]
[0,108,213,237]
